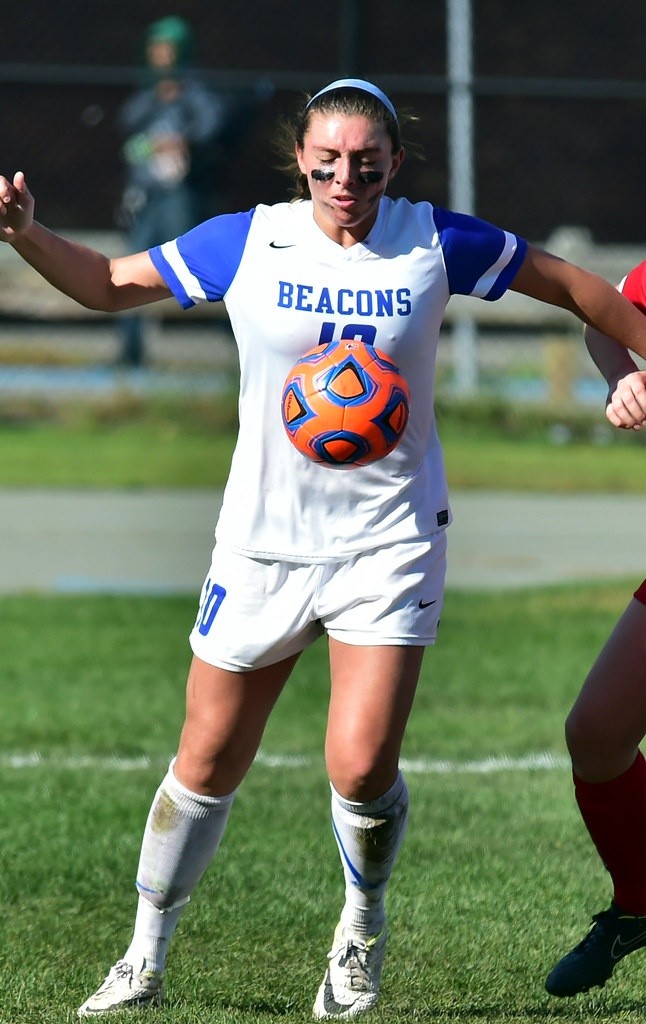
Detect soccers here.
[280,339,412,471]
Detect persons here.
[104,15,224,378]
[0,79,645,1017]
[544,259,645,1000]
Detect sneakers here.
[311,922,388,1020]
[545,910,646,996]
[77,957,167,1018]
